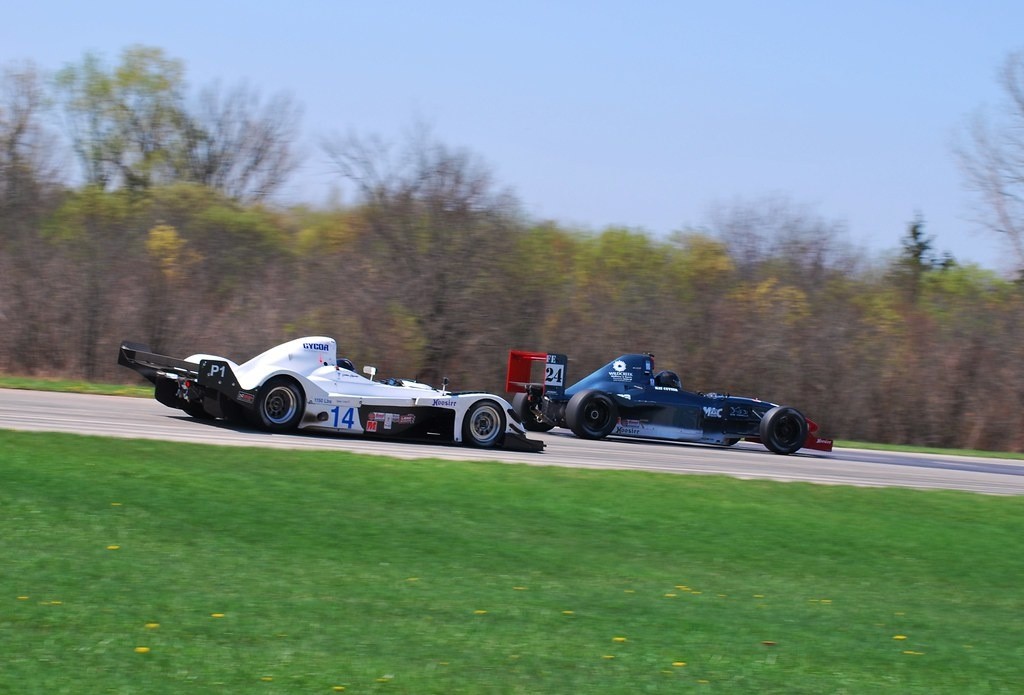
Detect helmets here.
[654,371,680,389]
[337,359,354,372]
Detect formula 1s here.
[116,333,548,453]
[505,345,833,456]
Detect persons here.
[337,357,358,375]
[656,369,683,389]
